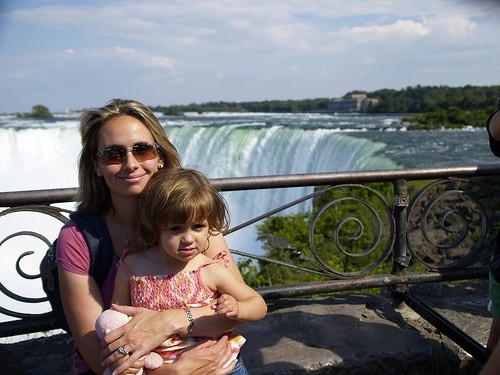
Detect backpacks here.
[40,211,113,338]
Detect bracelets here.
[182,306,195,338]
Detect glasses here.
[97,142,160,165]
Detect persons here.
[54,99,250,375]
[109,168,267,375]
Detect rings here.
[118,346,128,355]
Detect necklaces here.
[114,216,140,246]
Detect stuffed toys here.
[94,308,163,375]
